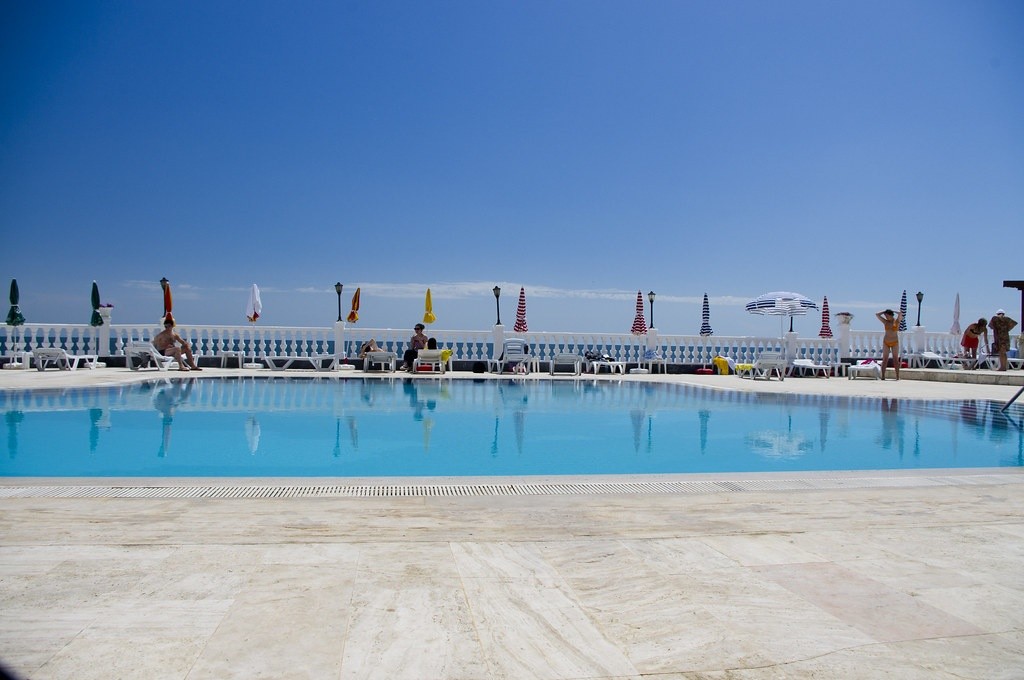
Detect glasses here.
[414,328,422,330]
[164,325,173,328]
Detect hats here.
[995,309,1005,313]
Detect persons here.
[404,379,436,421]
[153,319,203,371]
[400,323,437,373]
[358,339,383,360]
[876,308,904,380]
[989,309,1018,371]
[961,318,991,358]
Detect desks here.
[531,358,539,372]
[23,352,34,370]
[831,363,851,377]
[220,351,246,368]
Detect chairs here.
[921,352,978,370]
[549,353,582,376]
[848,360,883,380]
[412,349,453,374]
[582,353,627,376]
[34,348,94,372]
[792,358,832,378]
[736,350,788,381]
[985,357,1024,370]
[123,341,200,372]
[264,352,345,372]
[363,352,398,374]
[486,338,533,375]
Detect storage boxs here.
[899,361,908,368]
[1006,347,1019,358]
[435,365,440,371]
[416,365,432,371]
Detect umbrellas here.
[951,293,961,335]
[6,410,962,456]
[5,275,907,327]
